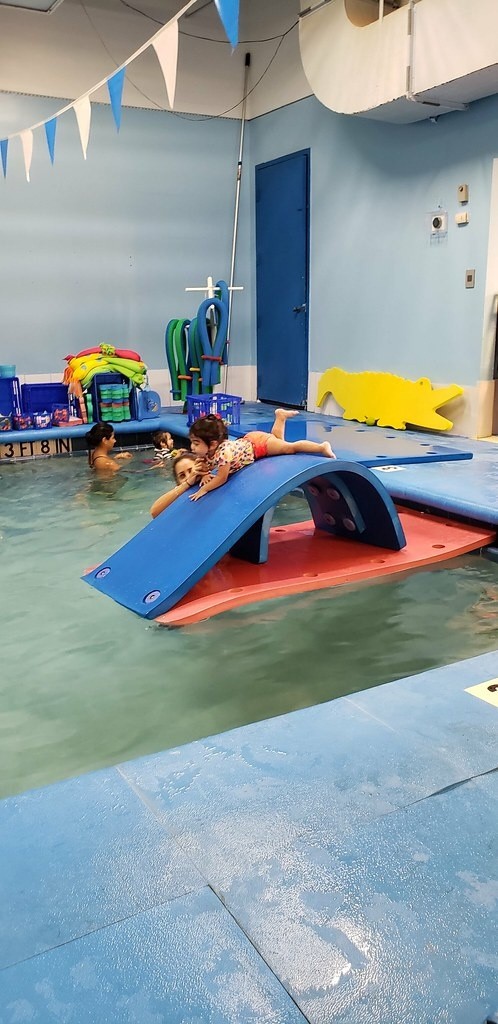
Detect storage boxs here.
[88,372,137,423]
[185,393,242,428]
[0,365,70,433]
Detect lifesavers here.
[165,280,231,402]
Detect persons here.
[83,423,164,472]
[149,409,337,519]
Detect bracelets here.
[174,486,179,496]
[185,479,191,486]
